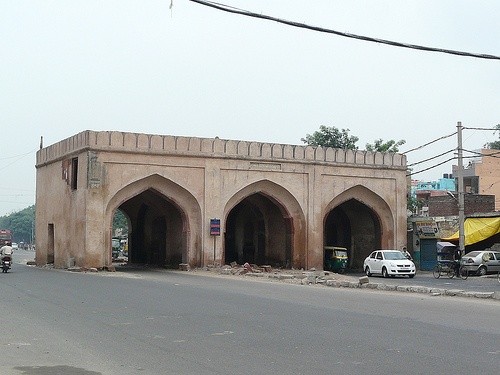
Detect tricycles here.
[433,241,469,280]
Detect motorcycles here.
[1,249,14,272]
[324,246,348,273]
[111,237,121,259]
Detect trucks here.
[0,229,13,254]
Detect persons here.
[453,246,462,278]
[0,241,14,269]
[401,246,413,260]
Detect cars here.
[461,251,500,276]
[363,249,416,278]
[12,243,18,250]
